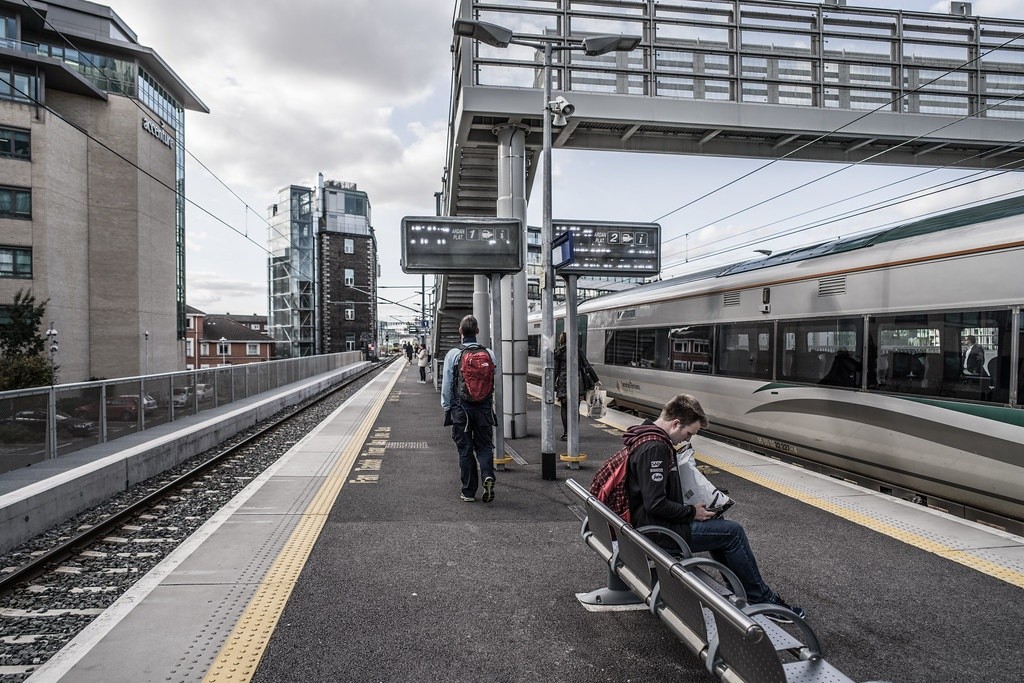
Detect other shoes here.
[560,434,567,441]
[417,380,426,384]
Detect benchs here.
[566,478,858,683]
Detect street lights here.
[45,320,58,460]
[453,14,643,477]
[142,329,150,376]
[219,336,228,366]
[415,290,431,330]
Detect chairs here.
[720,350,1009,403]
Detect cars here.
[165,386,196,408]
[189,384,214,402]
[0,407,96,439]
[68,395,158,421]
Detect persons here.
[406,342,413,364]
[416,344,428,384]
[414,343,418,358]
[441,314,497,504]
[625,395,805,625]
[553,332,602,441]
[403,341,407,349]
[963,335,987,377]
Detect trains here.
[526,190,1024,525]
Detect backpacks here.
[454,344,495,403]
[588,435,675,541]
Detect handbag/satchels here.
[579,352,595,389]
[675,442,736,520]
[428,355,431,362]
[588,384,608,420]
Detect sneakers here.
[460,493,475,502]
[481,477,495,502]
[763,592,805,623]
